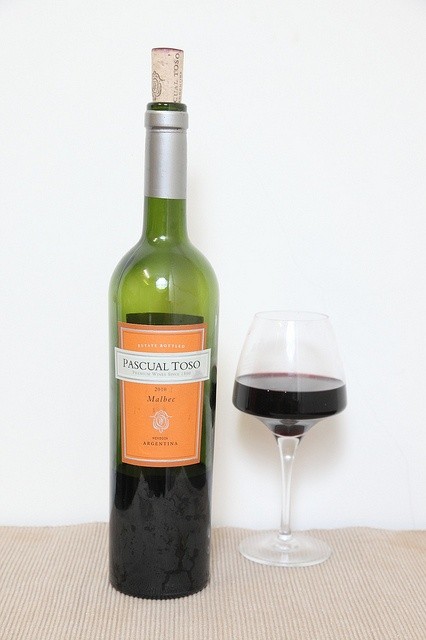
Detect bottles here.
[109,47,219,600]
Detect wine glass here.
[233,310,347,568]
